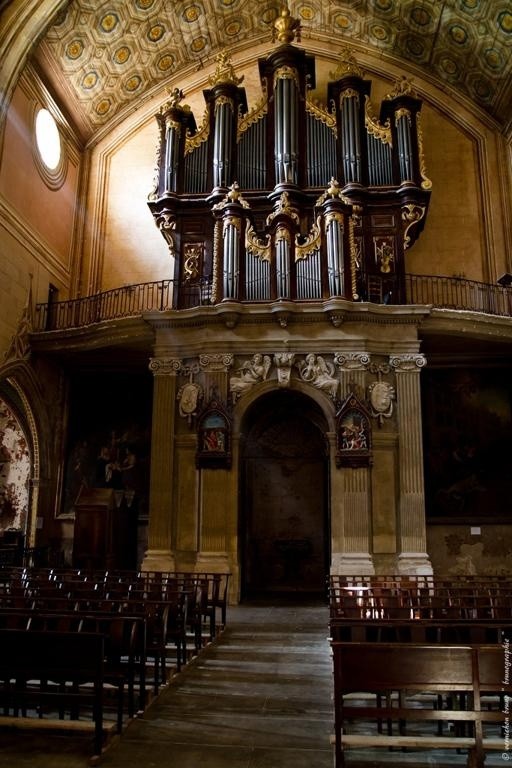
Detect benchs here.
[1,566,233,765]
[326,572,511,767]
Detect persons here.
[303,353,340,399]
[229,354,265,398]
[103,444,136,485]
